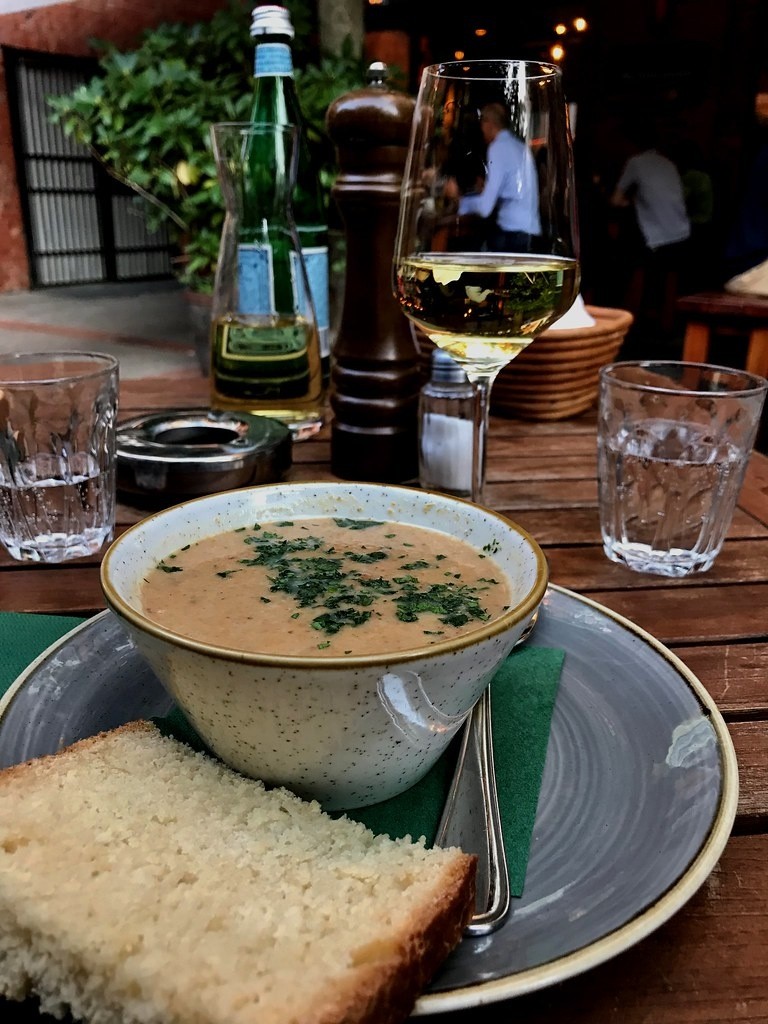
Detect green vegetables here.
[156,515,513,653]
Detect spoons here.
[432,606,538,936]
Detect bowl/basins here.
[99,481,548,811]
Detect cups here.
[0,351,119,564]
[598,360,768,578]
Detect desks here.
[678,292,768,390]
[1,360,766,1024]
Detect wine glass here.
[392,59,578,504]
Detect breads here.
[0,718,481,1024]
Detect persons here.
[595,138,691,310]
[457,103,542,252]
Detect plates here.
[0,583,739,1024]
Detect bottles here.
[215,5,331,390]
[418,349,489,503]
[211,122,326,440]
